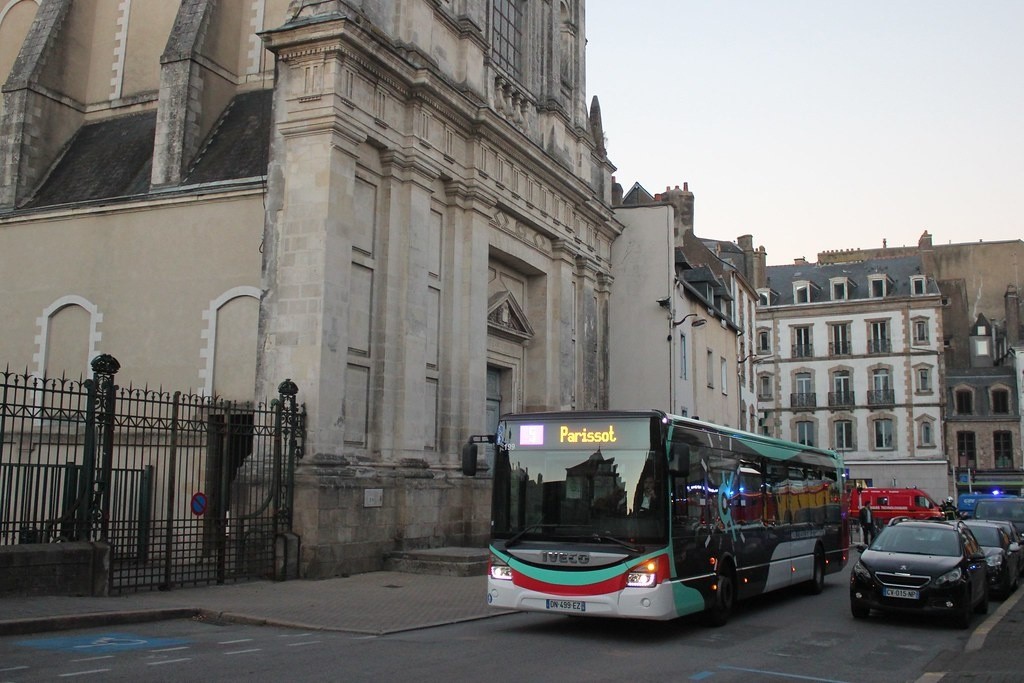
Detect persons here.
[941,496,962,521]
[858,500,877,546]
[631,475,660,514]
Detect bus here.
[462,408,848,628]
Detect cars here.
[955,493,1024,600]
[850,516,987,629]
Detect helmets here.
[946,495,953,503]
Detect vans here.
[850,486,944,525]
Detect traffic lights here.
[955,467,960,481]
[972,469,976,483]
